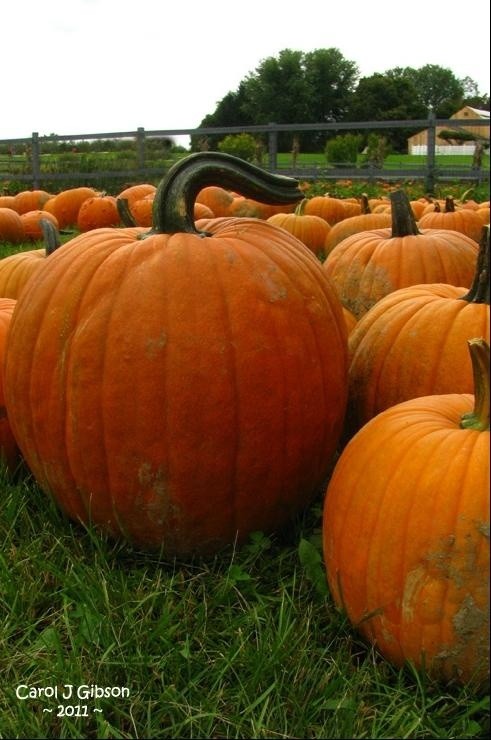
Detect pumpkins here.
[0,151,490,682]
